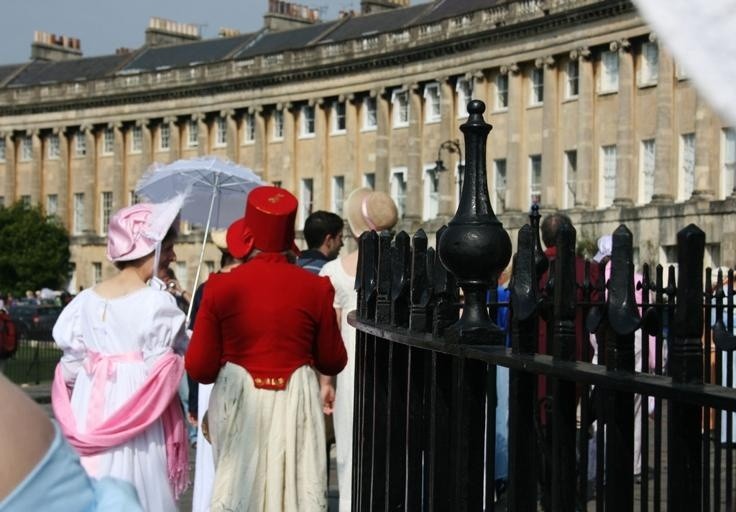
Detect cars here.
[0,291,75,357]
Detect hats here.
[227,186,303,261]
[210,226,228,253]
[107,195,187,270]
[593,236,613,264]
[344,187,399,240]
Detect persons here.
[185,184,348,511]
[482,264,514,507]
[586,234,659,504]
[709,270,736,450]
[322,186,399,512]
[701,266,731,439]
[294,210,345,490]
[166,278,192,303]
[535,212,604,425]
[135,154,273,320]
[187,226,244,512]
[0,285,72,308]
[52,200,190,511]
[157,224,188,312]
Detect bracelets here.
[175,288,185,299]
